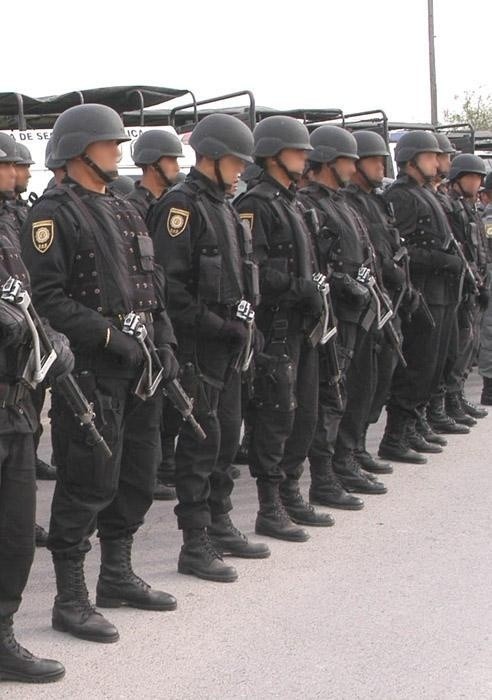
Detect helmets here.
[350,130,391,161]
[132,130,186,166]
[395,130,455,163]
[44,104,132,169]
[448,153,488,180]
[188,113,255,168]
[238,115,314,182]
[0,132,35,166]
[304,125,360,164]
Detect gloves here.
[304,289,325,315]
[446,253,463,275]
[341,273,371,308]
[106,326,146,368]
[405,289,421,315]
[47,331,77,383]
[251,323,266,355]
[0,299,32,352]
[155,344,180,380]
[216,318,247,346]
[476,292,491,313]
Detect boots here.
[0,611,66,683]
[379,390,488,464]
[151,478,177,501]
[51,530,177,644]
[256,446,393,544]
[33,522,53,548]
[481,376,492,404]
[34,458,61,480]
[178,511,270,583]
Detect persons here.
[1,102,490,685]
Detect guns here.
[302,273,349,413]
[232,300,258,402]
[356,264,411,371]
[442,231,480,298]
[394,249,414,320]
[121,312,209,442]
[0,276,112,458]
